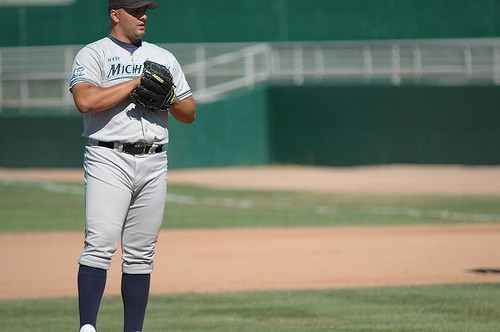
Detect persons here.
[67,0,197,331]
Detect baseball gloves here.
[127,60,175,112]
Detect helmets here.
[107,0,158,13]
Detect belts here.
[98,141,163,154]
